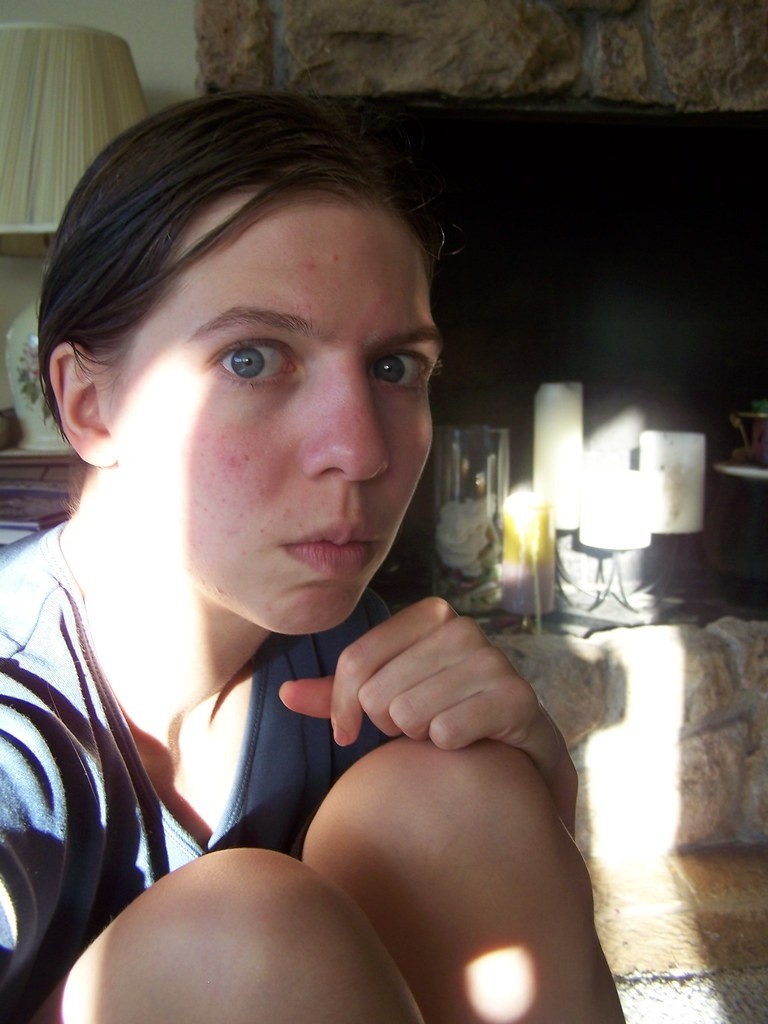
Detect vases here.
[4,299,79,452]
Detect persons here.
[0,92,624,1024]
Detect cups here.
[431,423,510,614]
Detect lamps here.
[0,19,150,251]
